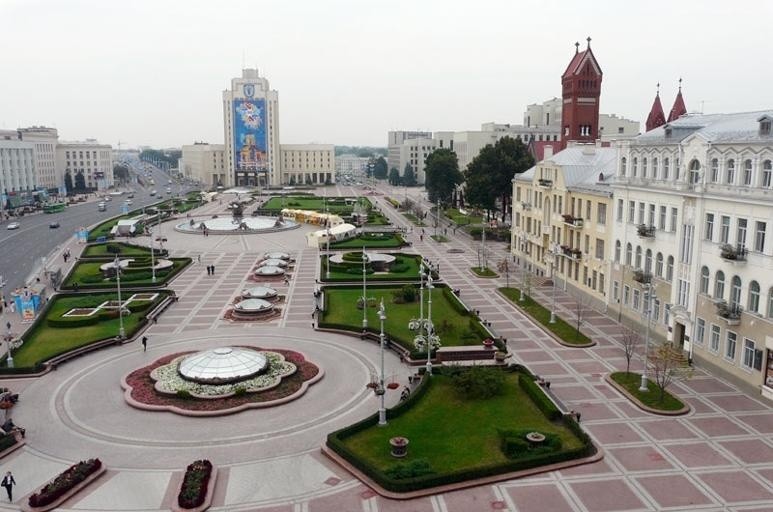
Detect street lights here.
[479,215,486,273]
[141,195,176,284]
[113,251,125,339]
[638,280,660,393]
[375,297,388,425]
[358,244,371,329]
[423,268,435,375]
[436,199,441,239]
[517,231,527,301]
[417,260,426,354]
[0,275,14,367]
[545,244,560,325]
[367,159,377,194]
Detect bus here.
[43,202,64,214]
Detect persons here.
[142,336,147,351]
[1,471,15,501]
[67,252,70,261]
[9,298,14,313]
[211,264,214,273]
[3,295,7,307]
[207,265,210,274]
[3,418,13,432]
[63,253,67,262]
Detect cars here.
[49,221,59,228]
[6,221,20,231]
[98,165,198,211]
